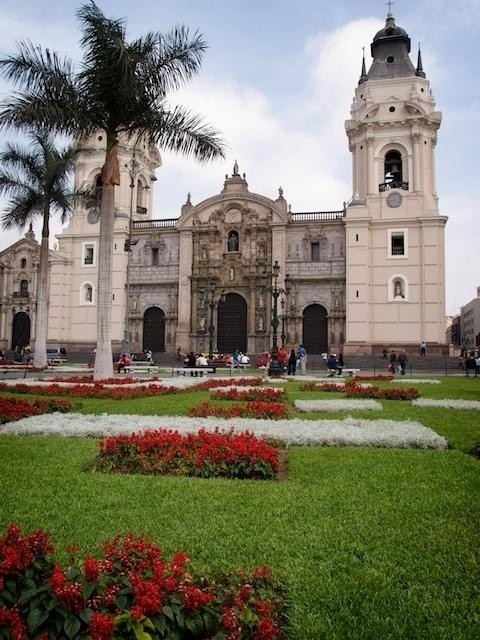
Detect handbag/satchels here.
[397,358,402,361]
[388,364,392,368]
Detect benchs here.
[116,361,160,374]
[328,368,361,377]
[225,362,251,371]
[170,367,214,376]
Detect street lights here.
[120,146,157,361]
[260,260,293,376]
[198,278,226,366]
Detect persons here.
[382,347,409,378]
[456,353,480,378]
[229,233,238,251]
[173,345,250,378]
[0,344,33,361]
[419,340,427,357]
[268,345,345,377]
[117,348,154,373]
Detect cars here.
[27,347,68,363]
